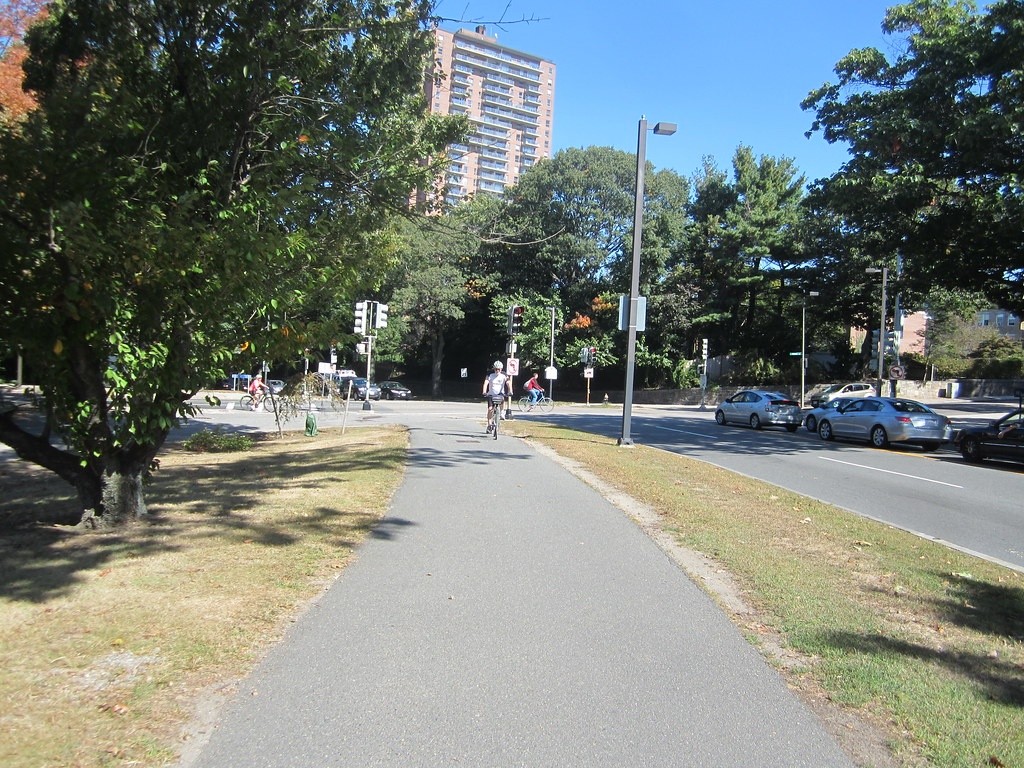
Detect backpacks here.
[523,377,534,390]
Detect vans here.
[811,383,876,410]
[324,370,357,389]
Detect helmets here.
[493,361,503,369]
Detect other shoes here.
[487,426,492,434]
[500,409,506,420]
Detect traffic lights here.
[353,299,390,336]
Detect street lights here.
[510,307,523,335]
[801,290,820,410]
[617,114,677,447]
[590,347,598,364]
[866,267,887,398]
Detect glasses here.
[494,368,501,371]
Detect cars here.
[714,391,803,432]
[955,409,1024,465]
[341,377,411,400]
[817,397,953,450]
[267,380,286,393]
[804,398,862,432]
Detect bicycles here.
[484,394,512,440]
[240,388,277,412]
[518,390,554,413]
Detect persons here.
[249,374,269,410]
[527,373,544,408]
[482,360,513,433]
